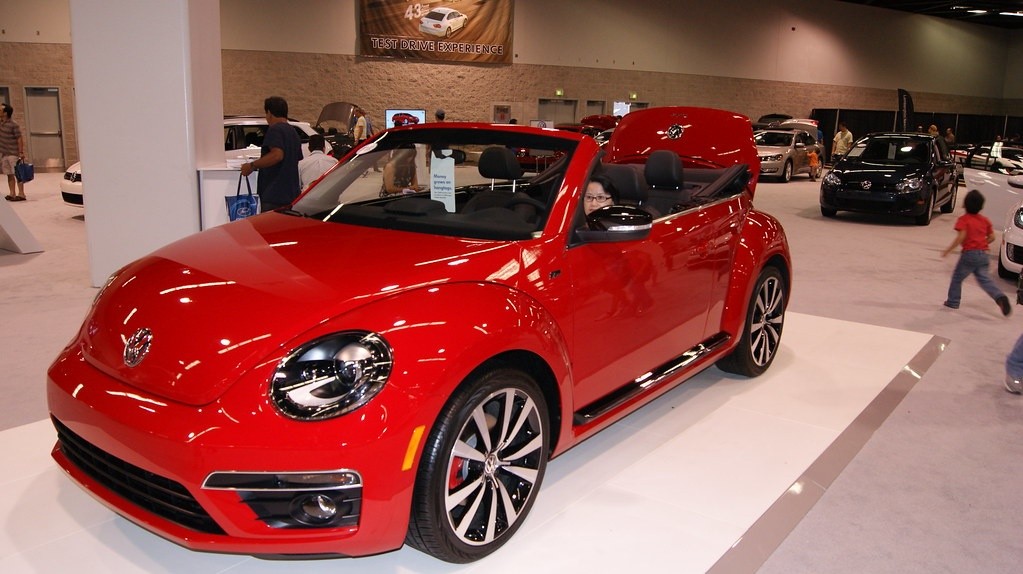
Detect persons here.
[381,144,421,195]
[353,108,374,145]
[818,130,824,145]
[928,125,939,135]
[806,148,820,181]
[991,136,1003,158]
[241,97,302,214]
[941,189,1011,316]
[583,175,622,217]
[426,109,445,175]
[299,134,338,190]
[505,119,517,153]
[0,103,27,202]
[1004,334,1023,392]
[944,128,955,145]
[832,122,853,164]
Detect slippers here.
[11,196,26,201]
[5,195,16,200]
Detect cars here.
[752,113,824,184]
[61,114,334,208]
[312,101,372,162]
[419,8,468,39]
[820,132,958,226]
[392,113,419,125]
[998,175,1023,282]
[508,114,618,168]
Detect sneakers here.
[1004,372,1022,393]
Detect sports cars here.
[46,106,795,566]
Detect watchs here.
[251,163,257,171]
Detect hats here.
[436,108,444,116]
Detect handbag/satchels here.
[14,157,34,182]
[225,167,261,222]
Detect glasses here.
[584,195,612,202]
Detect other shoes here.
[996,295,1010,315]
[944,300,948,305]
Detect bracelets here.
[19,152,23,153]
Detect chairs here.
[644,150,681,212]
[585,162,641,226]
[462,148,533,223]
[245,131,258,146]
